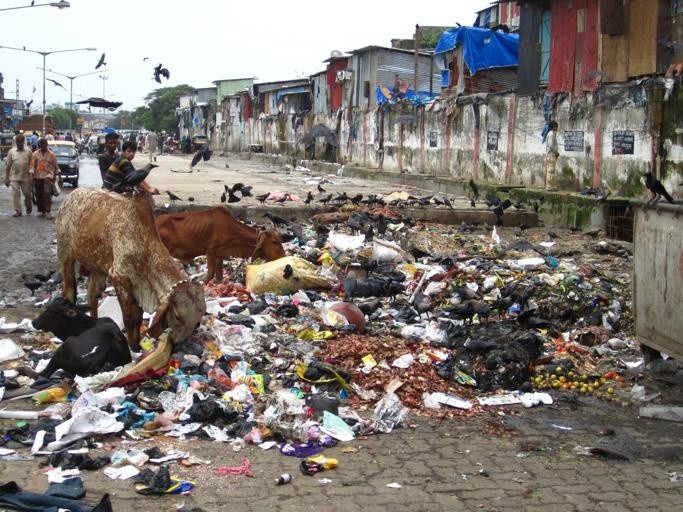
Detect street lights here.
[0,45,114,136]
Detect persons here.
[10,129,194,163]
[4,134,33,218]
[27,138,63,219]
[97,132,130,192]
[100,142,159,195]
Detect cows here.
[152,203,285,284]
[53,186,206,352]
[12,293,131,383]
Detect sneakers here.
[12,205,52,217]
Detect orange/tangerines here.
[530,366,614,394]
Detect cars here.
[0,131,41,161]
[47,141,78,188]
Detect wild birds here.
[188,144,213,167]
[94,52,107,70]
[641,171,674,207]
[296,120,336,150]
[152,61,169,83]
[164,189,180,204]
[187,197,195,204]
[217,179,604,331]
[74,98,123,111]
[46,78,65,90]
